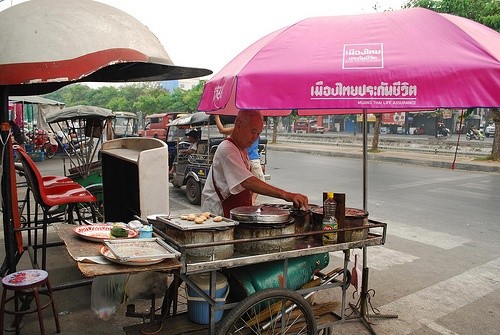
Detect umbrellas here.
[198,7,500,318]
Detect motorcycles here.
[157,111,270,205]
[112,111,138,138]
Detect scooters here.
[466,130,484,141]
[437,128,451,138]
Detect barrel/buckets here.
[185,270,230,324]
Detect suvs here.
[139,112,185,140]
[294,119,324,134]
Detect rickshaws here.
[17,104,120,227]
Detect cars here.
[485,123,495,136]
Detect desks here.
[101,136,169,222]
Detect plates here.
[73,225,137,242]
[98,244,165,265]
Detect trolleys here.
[15,201,388,335]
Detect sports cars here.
[42,130,94,159]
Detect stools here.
[0,269,61,335]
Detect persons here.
[213,114,265,205]
[169,130,202,175]
[201,109,308,219]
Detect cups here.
[140,229,153,239]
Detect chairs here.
[12,145,96,270]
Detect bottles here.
[322,192,338,246]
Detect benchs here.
[203,135,268,156]
